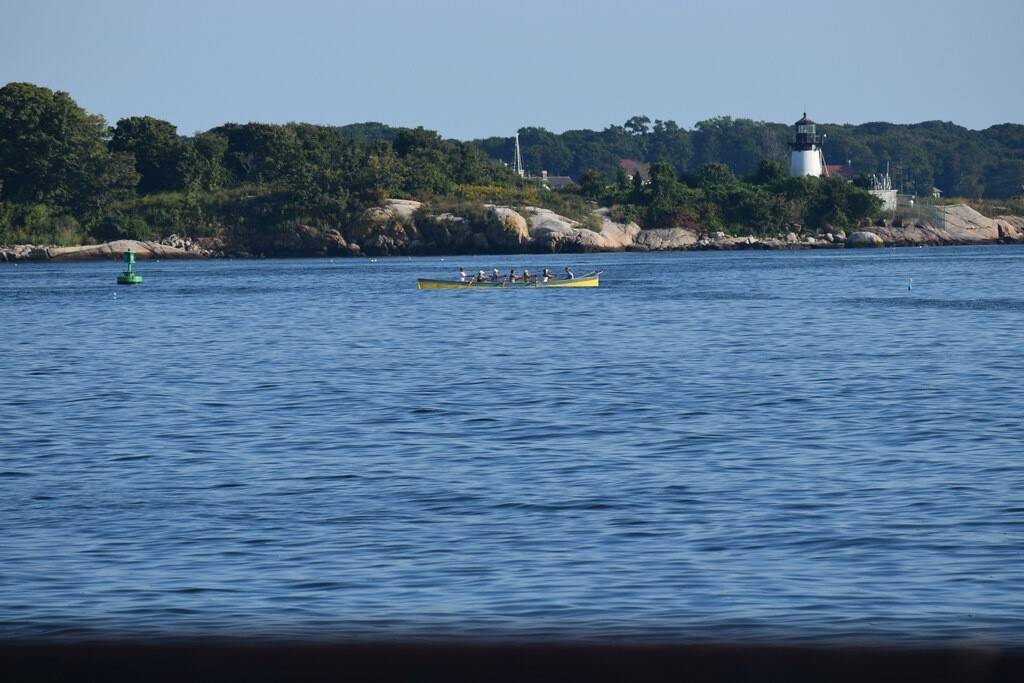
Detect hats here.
[543,269,548,272]
[565,267,570,271]
[479,270,484,273]
[493,269,498,273]
[524,270,529,273]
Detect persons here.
[459,266,575,284]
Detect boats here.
[417,269,604,291]
